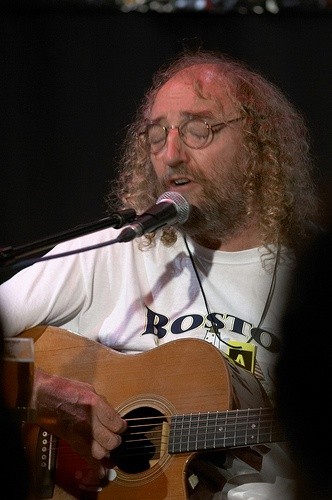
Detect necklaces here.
[181,225,282,375]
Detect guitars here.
[12,324,293,500]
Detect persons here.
[0,55,318,500]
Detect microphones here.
[118,191,191,243]
